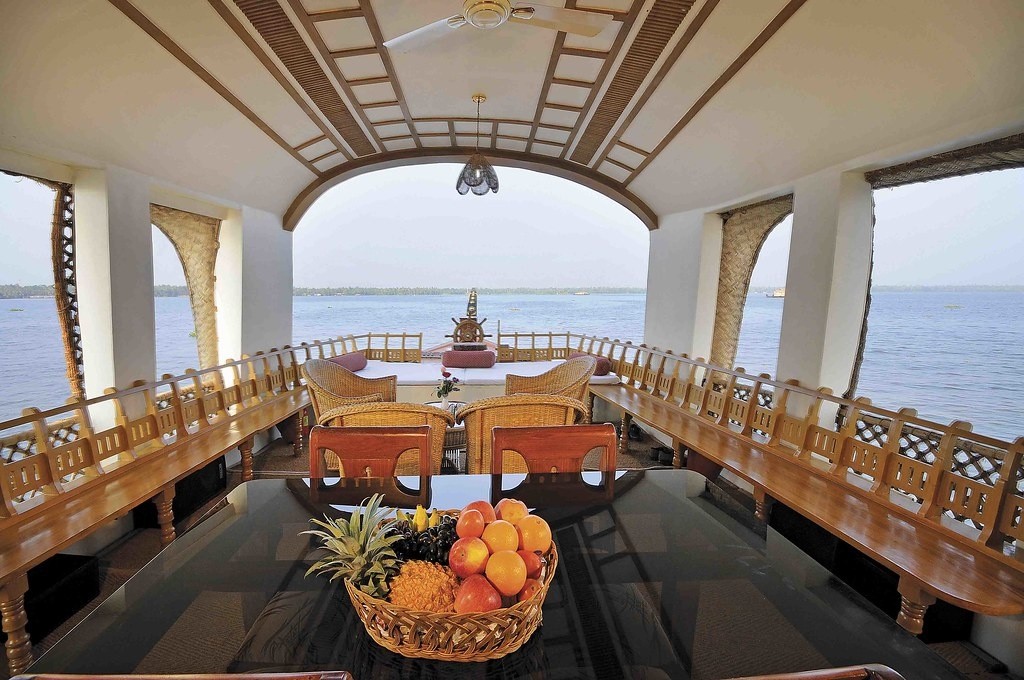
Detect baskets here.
[344,508,559,662]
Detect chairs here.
[455,394,589,475]
[301,359,396,424]
[490,423,616,475]
[316,401,455,474]
[504,355,597,406]
[309,425,432,477]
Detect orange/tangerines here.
[481,520,519,554]
[514,515,552,555]
[484,550,528,596]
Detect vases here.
[440,394,448,410]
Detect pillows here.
[565,351,612,377]
[443,351,495,367]
[329,352,368,372]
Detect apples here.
[449,498,542,614]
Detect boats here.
[574,292,590,295]
[509,308,520,310]
[9,307,23,311]
[328,306,332,308]
[766,289,785,298]
[189,331,196,337]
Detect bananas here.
[396,504,440,532]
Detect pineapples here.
[298,493,461,613]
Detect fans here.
[380,0,614,54]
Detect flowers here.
[429,367,461,398]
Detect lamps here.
[457,93,500,195]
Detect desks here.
[422,400,474,474]
[14,469,969,679]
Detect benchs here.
[498,322,1023,637]
[0,329,420,674]
